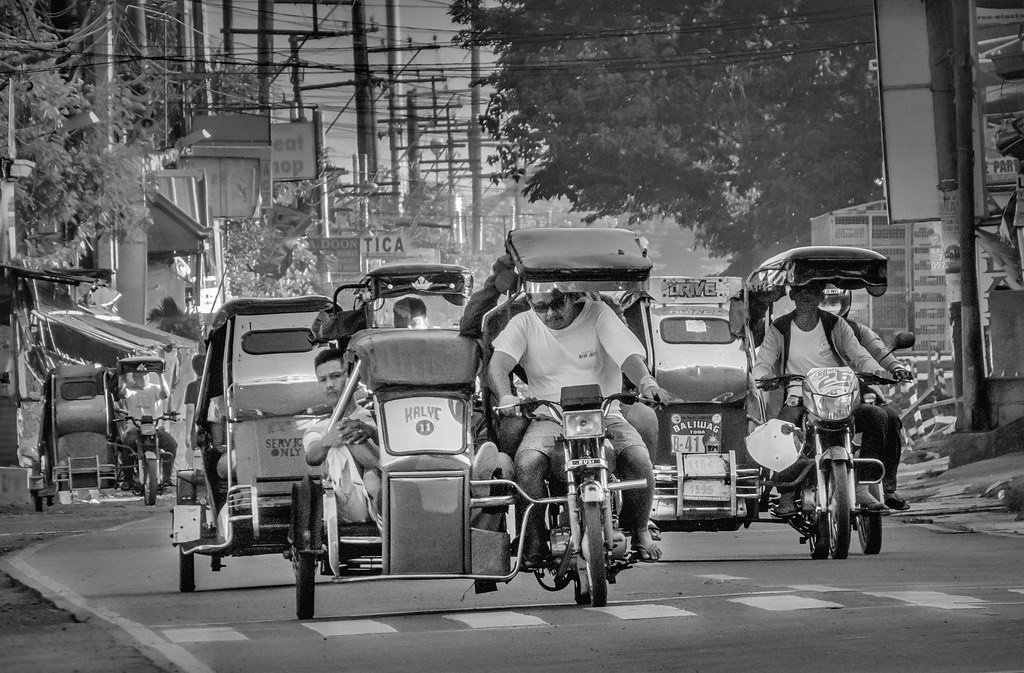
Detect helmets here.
[818,284,852,318]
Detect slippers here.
[858,502,891,513]
[775,491,796,516]
[631,543,662,562]
[520,545,551,568]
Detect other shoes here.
[471,442,500,497]
[121,481,130,490]
[647,518,662,541]
[163,479,176,487]
[885,492,910,510]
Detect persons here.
[393,297,430,330]
[489,282,668,573]
[301,346,498,538]
[752,279,914,518]
[183,353,206,448]
[119,369,179,492]
[207,356,239,480]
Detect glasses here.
[820,299,839,307]
[791,284,822,295]
[530,295,566,313]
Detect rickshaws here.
[25,354,183,513]
[168,293,351,595]
[621,247,921,564]
[284,226,654,620]
